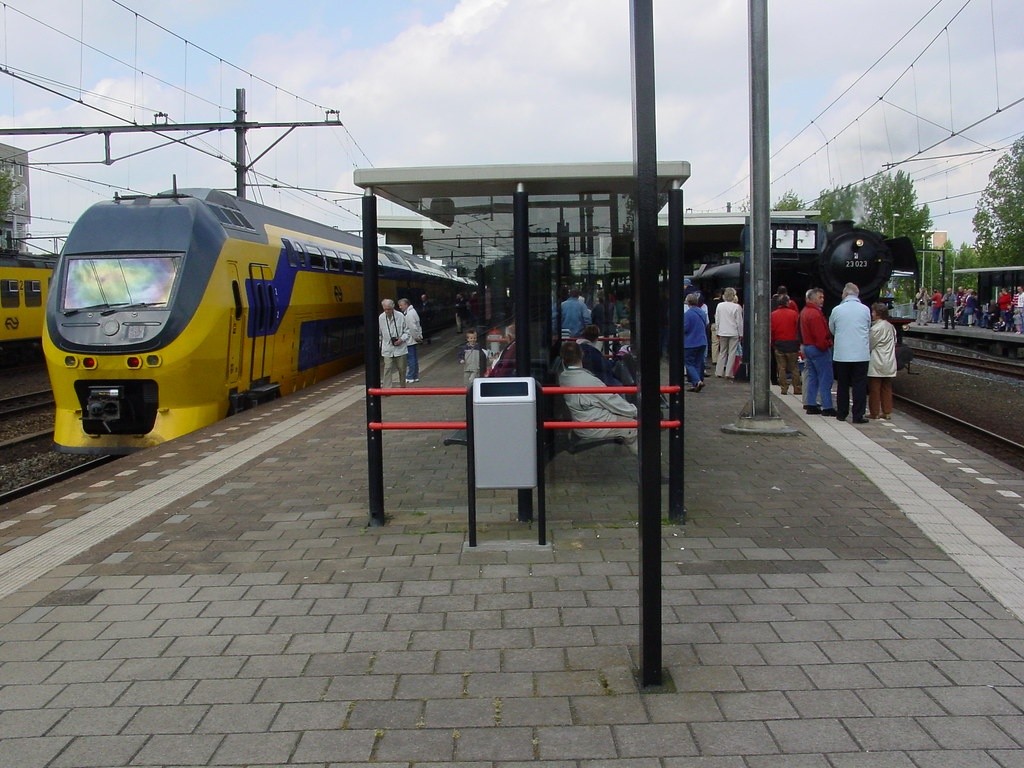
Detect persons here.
[455,294,465,333]
[380,298,409,389]
[398,298,423,382]
[485,324,517,377]
[550,280,1024,484]
[420,294,435,345]
[457,330,480,389]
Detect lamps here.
[430,198,454,227]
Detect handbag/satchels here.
[964,307,974,315]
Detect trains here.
[0,174,477,455]
[611,220,920,376]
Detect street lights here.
[892,213,900,238]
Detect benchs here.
[552,358,625,453]
[443,420,467,446]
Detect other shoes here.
[661,465,669,482]
[880,413,891,419]
[865,414,879,419]
[406,378,415,384]
[415,378,419,382]
[952,327,955,329]
[943,327,948,329]
[837,416,845,421]
[803,405,807,409]
[821,408,837,416]
[969,324,973,328]
[686,386,695,392]
[853,418,869,423]
[695,382,705,393]
[1015,331,1022,335]
[806,406,823,414]
